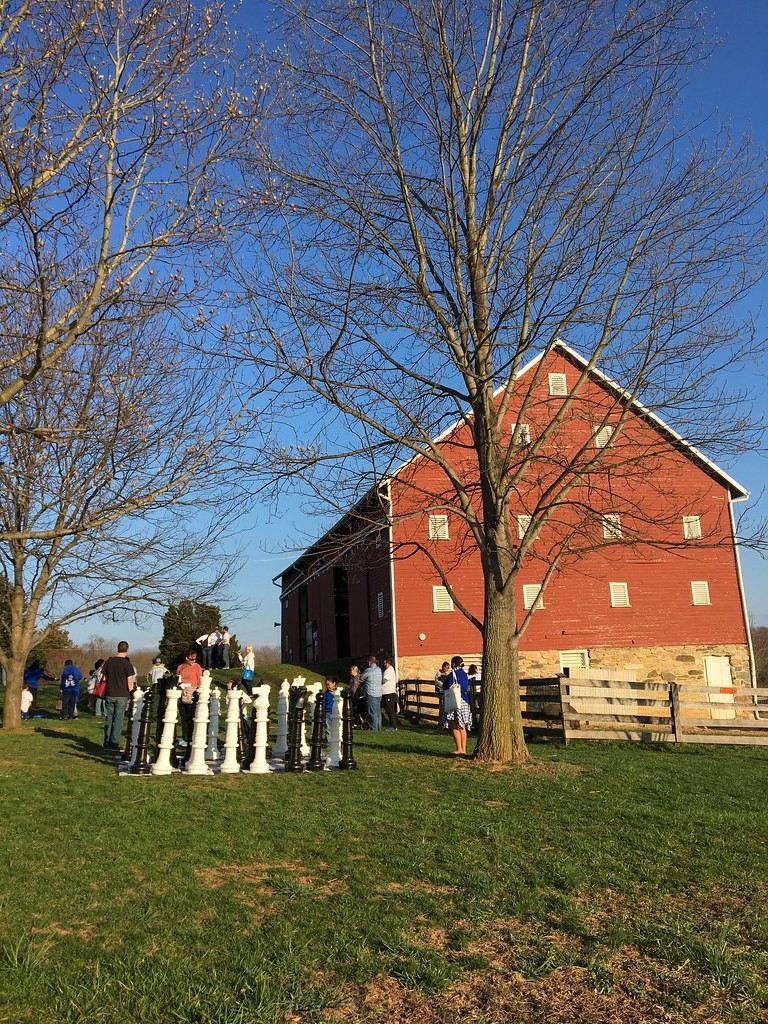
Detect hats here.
[154,658,162,663]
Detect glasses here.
[214,628,219,630]
[187,657,197,661]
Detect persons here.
[148,658,166,695]
[225,677,248,747]
[237,645,256,697]
[435,661,451,728]
[176,650,205,747]
[467,663,481,693]
[196,626,218,670]
[440,655,472,755]
[323,677,343,748]
[218,626,230,669]
[21,683,33,720]
[349,666,367,729]
[73,681,80,719]
[87,670,96,708]
[125,656,137,688]
[23,658,60,717]
[99,640,135,752]
[59,660,82,719]
[210,626,222,670]
[381,657,399,731]
[359,656,383,732]
[92,659,108,717]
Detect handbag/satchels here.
[201,635,210,647]
[92,680,107,700]
[443,684,462,713]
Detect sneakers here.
[384,726,398,731]
